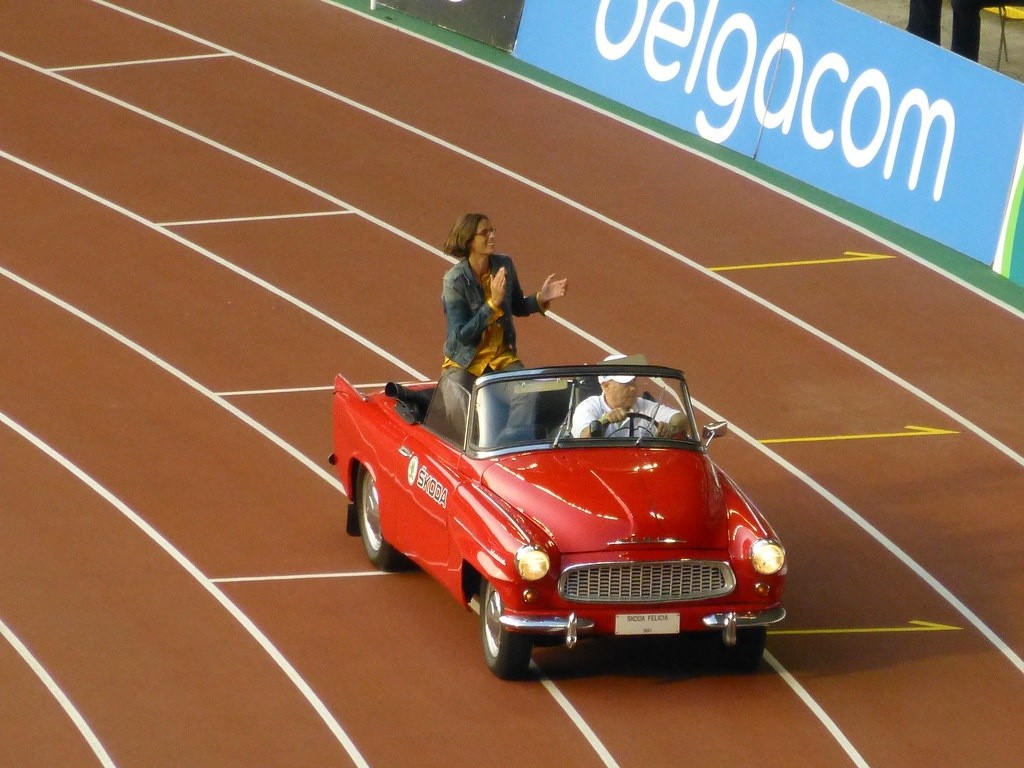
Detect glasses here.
[472,228,496,236]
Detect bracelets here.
[599,413,610,427]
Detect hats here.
[598,353,648,385]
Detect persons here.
[571,355,690,438]
[441,213,567,448]
[907,0,1024,63]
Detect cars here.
[321,355,792,681]
